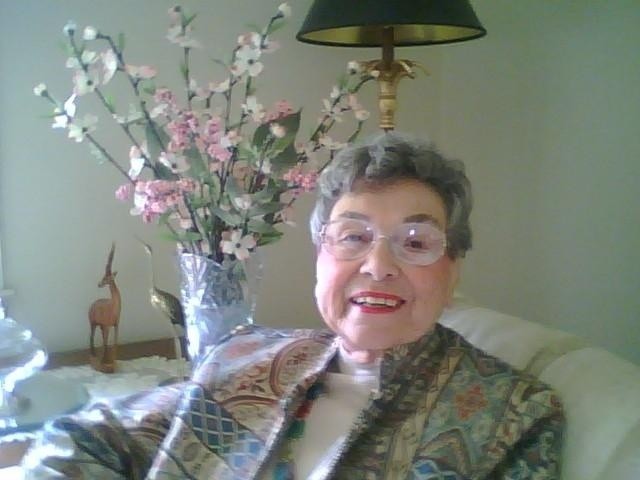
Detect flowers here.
[32,3,380,308]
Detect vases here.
[177,244,263,367]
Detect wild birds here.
[137,236,184,357]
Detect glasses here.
[316,216,453,267]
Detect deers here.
[88,243,123,356]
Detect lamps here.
[296,0,487,135]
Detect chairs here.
[436,304,640,480]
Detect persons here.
[20,131,565,480]
